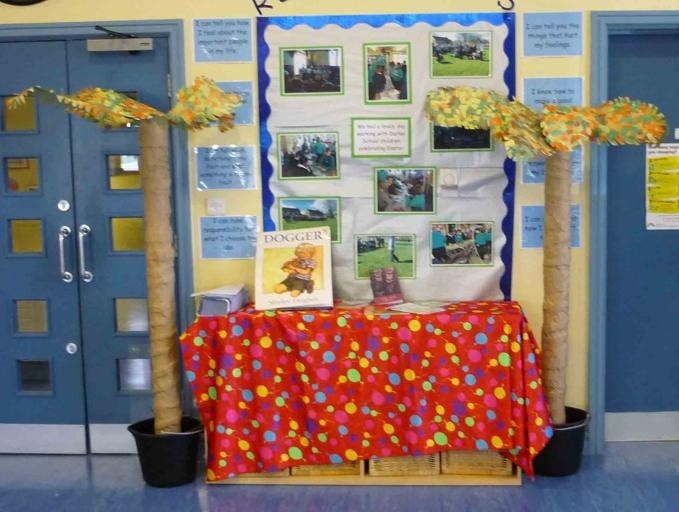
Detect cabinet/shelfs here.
[206,301,525,485]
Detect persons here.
[280,135,337,176]
[429,221,492,265]
[432,38,485,64]
[367,60,408,102]
[377,169,433,213]
[388,236,401,262]
[275,242,317,297]
